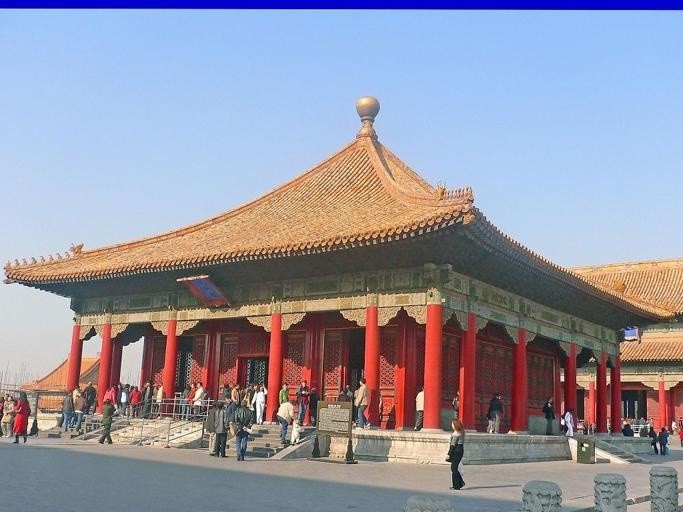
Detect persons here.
[620,417,682,455]
[336,384,352,425]
[206,394,254,460]
[541,396,555,435]
[97,399,116,445]
[413,386,424,430]
[564,407,574,436]
[275,380,317,445]
[221,383,268,425]
[451,390,459,419]
[62,381,96,433]
[103,382,166,419]
[444,419,465,490]
[180,382,205,421]
[486,392,503,434]
[353,378,384,430]
[0,391,31,443]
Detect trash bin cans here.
[576,437,596,464]
